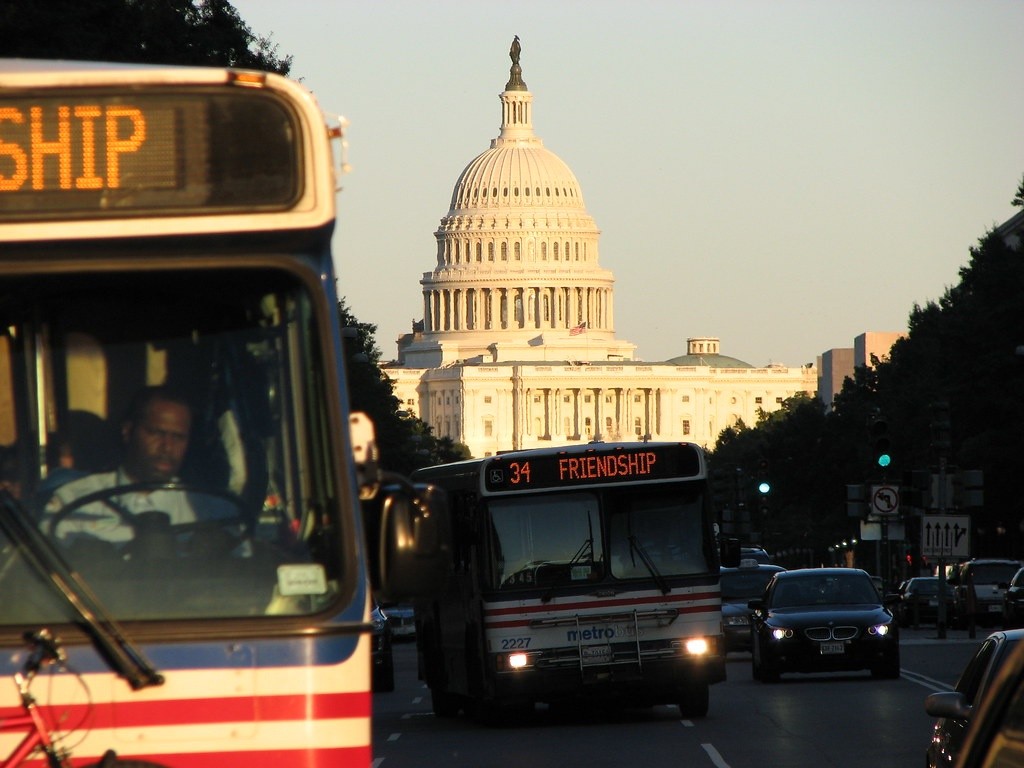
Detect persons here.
[40,397,253,560]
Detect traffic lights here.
[906,553,913,567]
[755,458,772,495]
[871,420,895,476]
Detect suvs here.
[716,543,773,590]
[946,558,1022,622]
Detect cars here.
[892,577,958,625]
[997,567,1024,629]
[923,625,1024,768]
[385,601,416,638]
[747,567,903,680]
[720,563,788,654]
[371,592,395,693]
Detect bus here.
[1,60,382,768]
[416,440,744,723]
